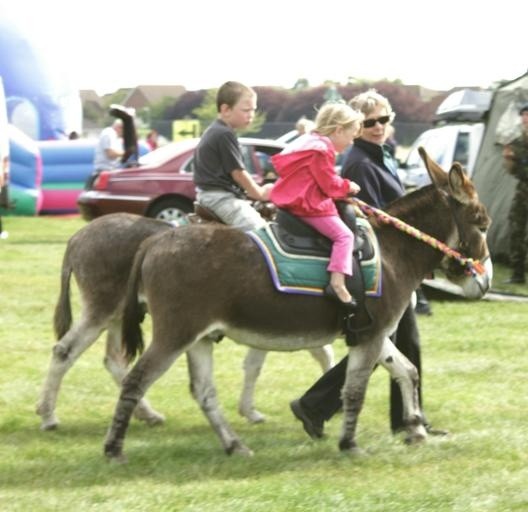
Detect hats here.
[519,104,528,115]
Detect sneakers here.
[503,277,525,283]
[394,424,447,435]
[290,398,328,438]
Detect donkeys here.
[32,211,339,434]
[102,146,496,469]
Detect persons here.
[293,118,314,136]
[191,81,275,231]
[269,100,366,310]
[502,104,527,286]
[287,87,450,445]
[69,118,157,175]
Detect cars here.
[77,136,290,229]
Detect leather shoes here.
[325,284,356,308]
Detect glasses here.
[363,116,389,127]
